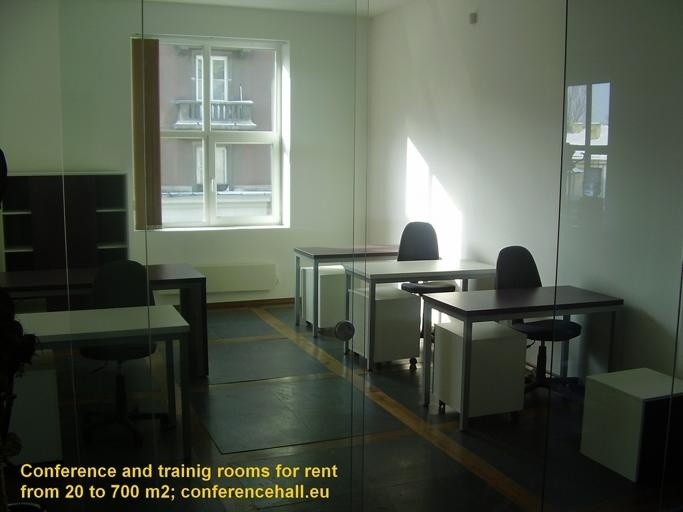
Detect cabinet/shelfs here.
[0,171,131,271]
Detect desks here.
[293,245,402,340]
[342,258,496,371]
[419,285,621,432]
[0,264,210,381]
[9,305,192,472]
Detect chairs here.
[495,246,579,413]
[75,258,168,448]
[380,223,456,373]
